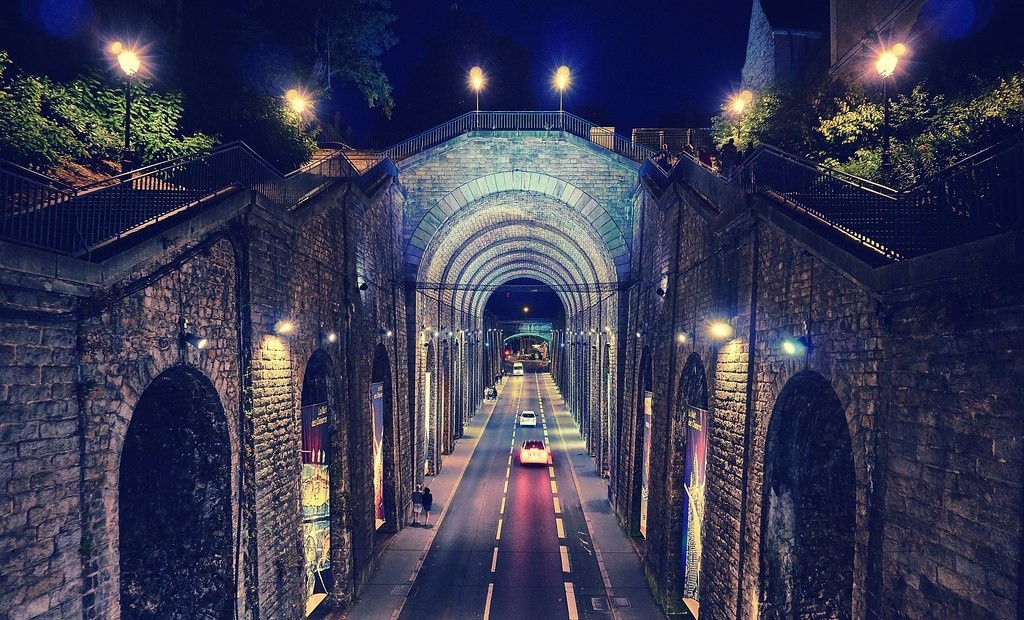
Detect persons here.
[411,485,433,525]
[651,138,756,178]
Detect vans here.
[513,362,524,376]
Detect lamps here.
[785,337,808,353]
[561,329,612,349]
[657,288,665,297]
[381,329,390,335]
[274,322,292,332]
[359,283,368,291]
[423,329,505,347]
[319,332,331,340]
[186,333,206,348]
[637,331,648,337]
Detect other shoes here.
[413,519,415,524]
[417,521,420,525]
[423,522,428,526]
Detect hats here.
[423,487,430,492]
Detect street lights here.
[733,99,745,140]
[875,54,901,179]
[116,50,141,189]
[554,73,569,127]
[470,72,486,130]
[291,94,306,139]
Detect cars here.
[518,410,539,428]
[520,439,548,466]
